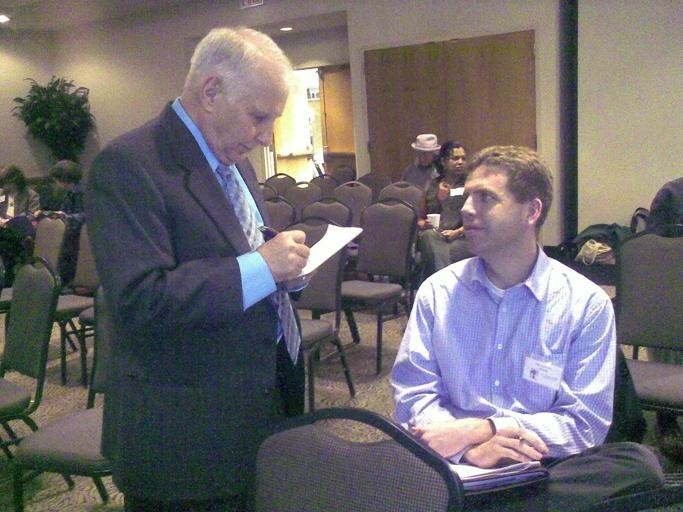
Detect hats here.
[411,133,441,152]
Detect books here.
[445,460,551,497]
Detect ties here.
[214,164,302,366]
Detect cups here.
[426,213,441,231]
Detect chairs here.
[247,403,463,512]
[0,255,59,454]
[614,225,682,416]
[13,406,119,510]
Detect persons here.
[0,162,42,287]
[413,141,474,273]
[401,133,444,188]
[382,139,670,510]
[78,21,311,510]
[33,159,89,282]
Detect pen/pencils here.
[260,226,278,238]
[520,437,523,441]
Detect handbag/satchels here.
[574,239,616,265]
